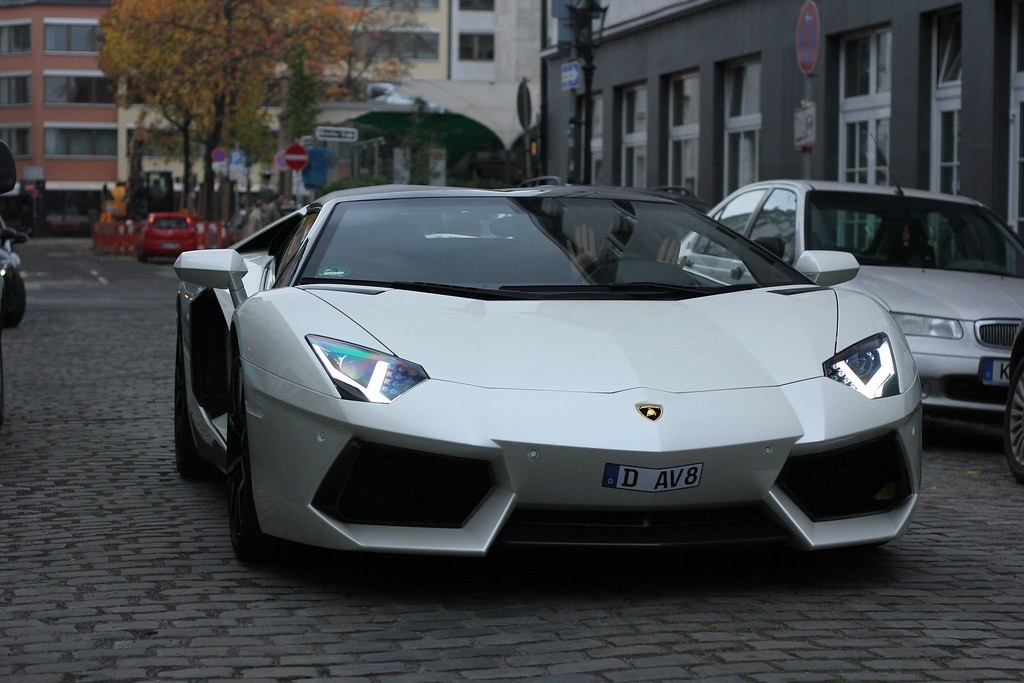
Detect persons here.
[542,199,682,287]
[241,194,284,239]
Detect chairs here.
[361,224,439,281]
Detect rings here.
[576,248,582,252]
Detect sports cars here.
[174,184,922,568]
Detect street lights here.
[565,0,609,185]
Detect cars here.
[520,175,1024,482]
[0,141,30,331]
[137,213,199,264]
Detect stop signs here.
[285,144,308,170]
